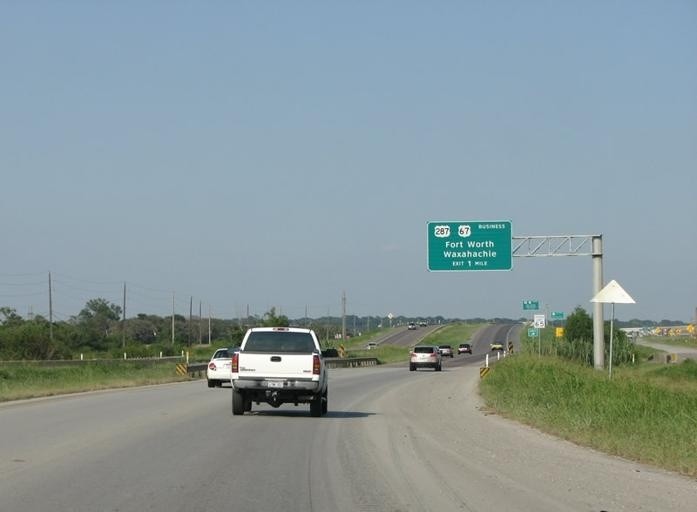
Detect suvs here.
[457,344,473,355]
[410,344,454,373]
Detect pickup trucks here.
[228,326,338,418]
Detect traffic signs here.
[534,315,544,328]
[426,219,513,272]
[527,328,537,337]
[550,311,563,319]
[522,300,538,310]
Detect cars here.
[490,342,503,351]
[408,322,416,330]
[367,342,377,351]
[419,320,427,327]
[206,347,237,388]
